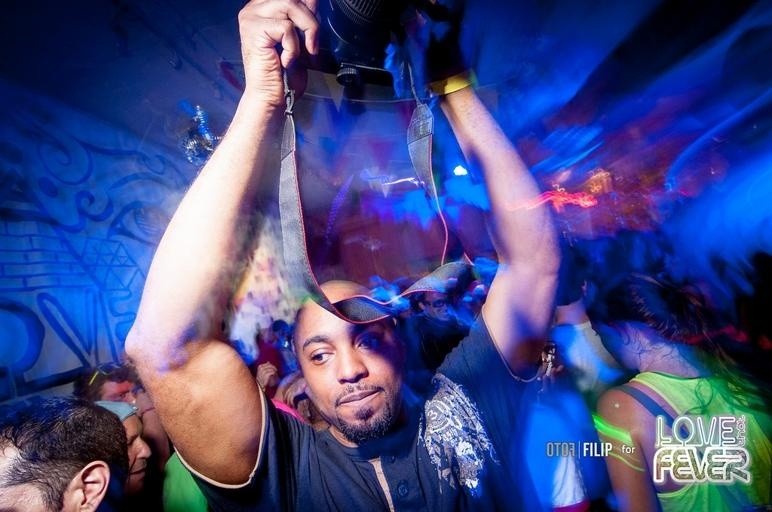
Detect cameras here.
[291,0,396,87]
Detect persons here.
[123,0,568,511]
[1,218,772,512]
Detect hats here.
[95,401,138,422]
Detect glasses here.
[90,363,125,384]
